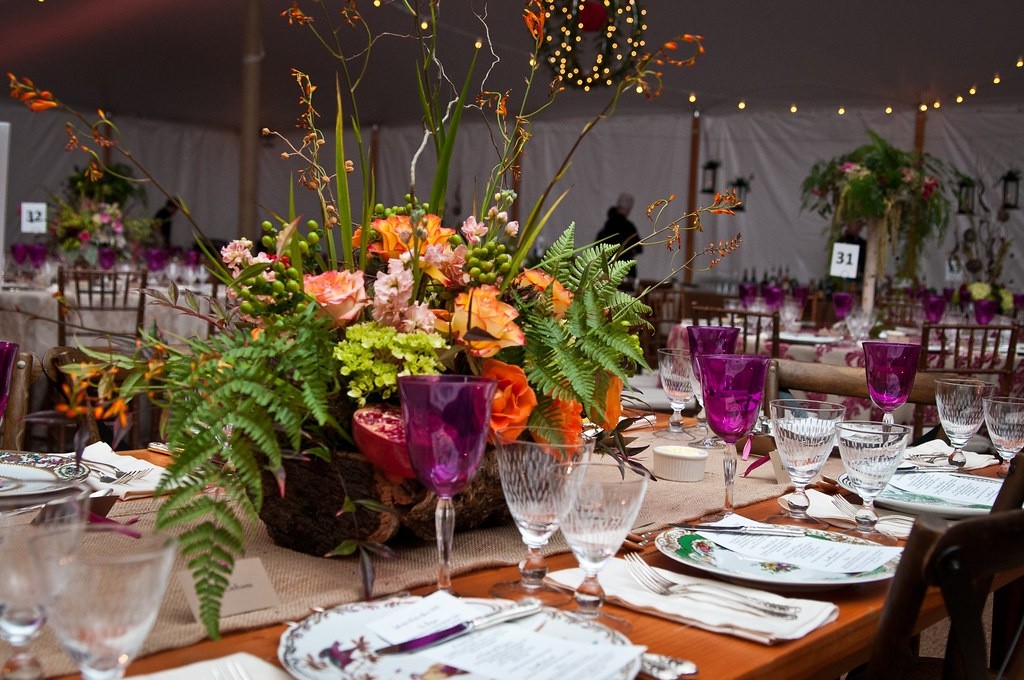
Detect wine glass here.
[8,241,211,287]
[654,284,1023,544]
[0,499,181,680]
[399,373,647,619]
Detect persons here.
[595,193,642,289]
[839,223,866,285]
[151,197,179,246]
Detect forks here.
[620,550,798,621]
[829,493,916,525]
[626,551,800,614]
[63,453,154,482]
[59,453,137,486]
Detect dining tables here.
[0,447,1024,680]
[0,280,239,450]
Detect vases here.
[246,415,510,559]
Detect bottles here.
[742,265,794,298]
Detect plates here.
[0,448,91,500]
[275,591,643,680]
[654,520,903,593]
[838,463,1024,512]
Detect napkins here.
[68,441,201,499]
[898,439,1000,472]
[544,558,840,644]
[774,488,912,539]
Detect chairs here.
[632,275,1024,439]
[0,265,228,454]
[845,448,1024,680]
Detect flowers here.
[39,158,174,278]
[795,130,968,276]
[0,0,746,642]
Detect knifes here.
[666,520,810,537]
[374,600,544,656]
